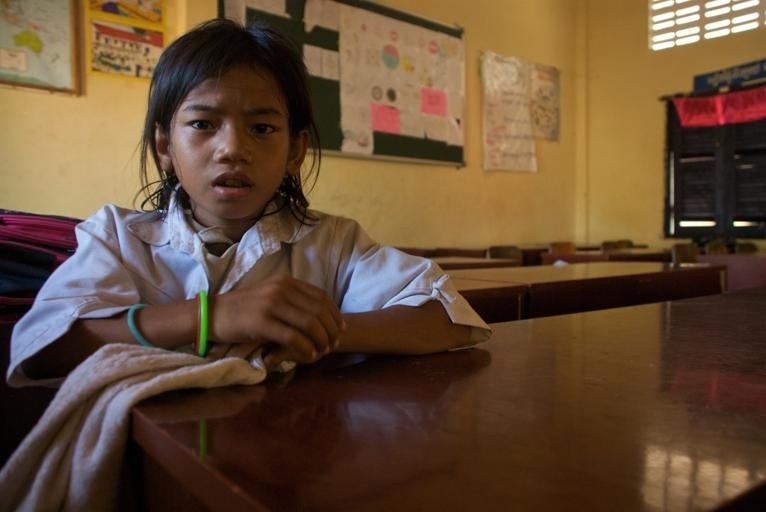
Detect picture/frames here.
[0,0,83,97]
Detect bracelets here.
[197,416,207,462]
[127,303,156,349]
[196,294,209,357]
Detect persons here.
[5,16,492,389]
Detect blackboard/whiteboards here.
[217,0,465,168]
